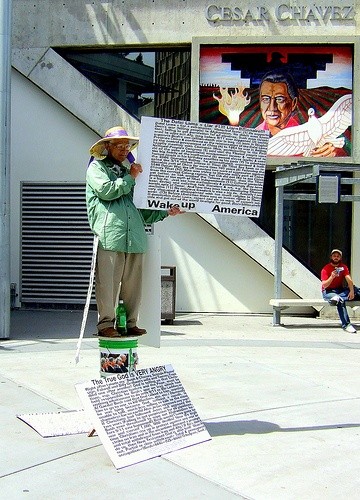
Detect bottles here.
[116,299,127,336]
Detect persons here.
[86,127,186,338]
[321,250,359,333]
[100,354,128,373]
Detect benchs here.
[270,298,360,326]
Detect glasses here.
[105,143,132,150]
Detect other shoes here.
[126,326,146,335]
[97,327,121,337]
[344,324,357,333]
[328,295,340,305]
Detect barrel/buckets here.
[98,335,138,378]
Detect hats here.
[331,249,343,256]
[89,126,140,160]
